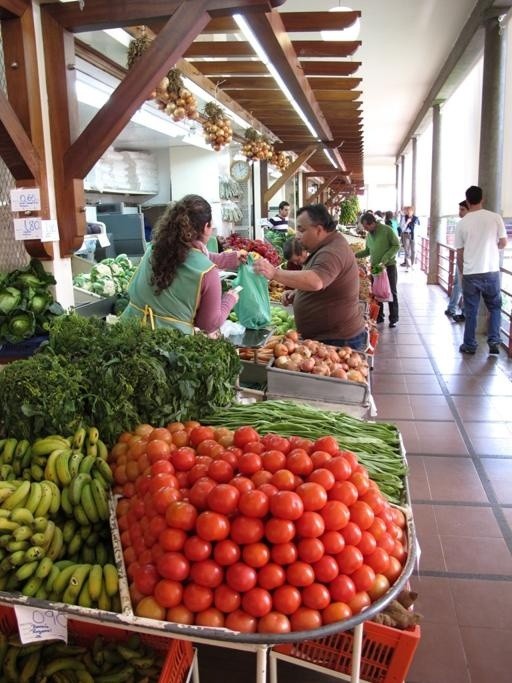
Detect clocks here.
[230,160,251,183]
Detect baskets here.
[0,603,195,683]
[271,579,421,683]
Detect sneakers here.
[444,310,455,317]
[459,344,476,354]
[488,342,500,354]
[453,315,465,322]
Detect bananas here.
[0,426,123,615]
[1,629,165,682]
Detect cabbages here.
[1,259,65,343]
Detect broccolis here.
[73,254,137,297]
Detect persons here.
[384,211,397,235]
[355,214,401,327]
[399,207,407,266]
[253,204,368,351]
[282,238,309,290]
[121,193,250,337]
[454,186,508,354]
[267,201,289,235]
[403,206,420,272]
[445,200,469,321]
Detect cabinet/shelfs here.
[196,222,371,419]
[72,190,170,323]
[0,419,422,683]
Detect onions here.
[243,139,292,171]
[273,329,369,384]
[148,77,200,122]
[203,114,233,152]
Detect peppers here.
[229,306,298,336]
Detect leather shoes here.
[389,320,397,328]
[377,317,384,323]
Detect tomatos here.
[109,420,409,634]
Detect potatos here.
[375,591,424,629]
